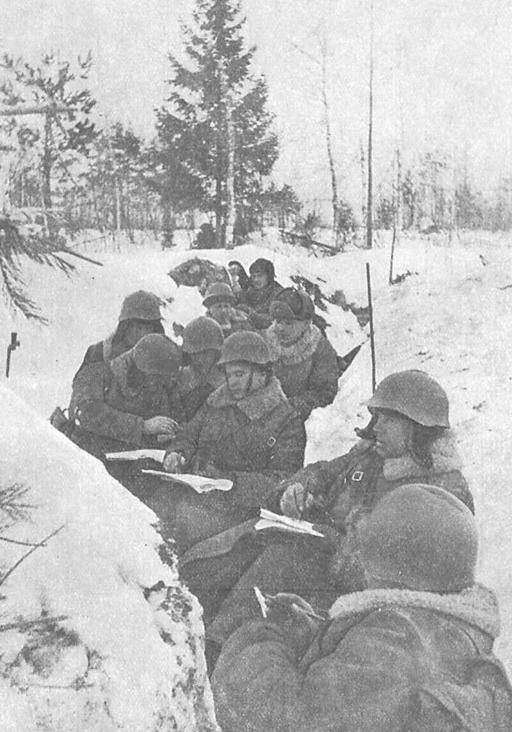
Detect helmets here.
[357,482,477,589]
[367,369,449,430]
[118,281,270,377]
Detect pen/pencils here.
[175,452,182,467]
[299,478,310,522]
[261,591,326,622]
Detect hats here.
[270,287,314,320]
[249,258,274,274]
